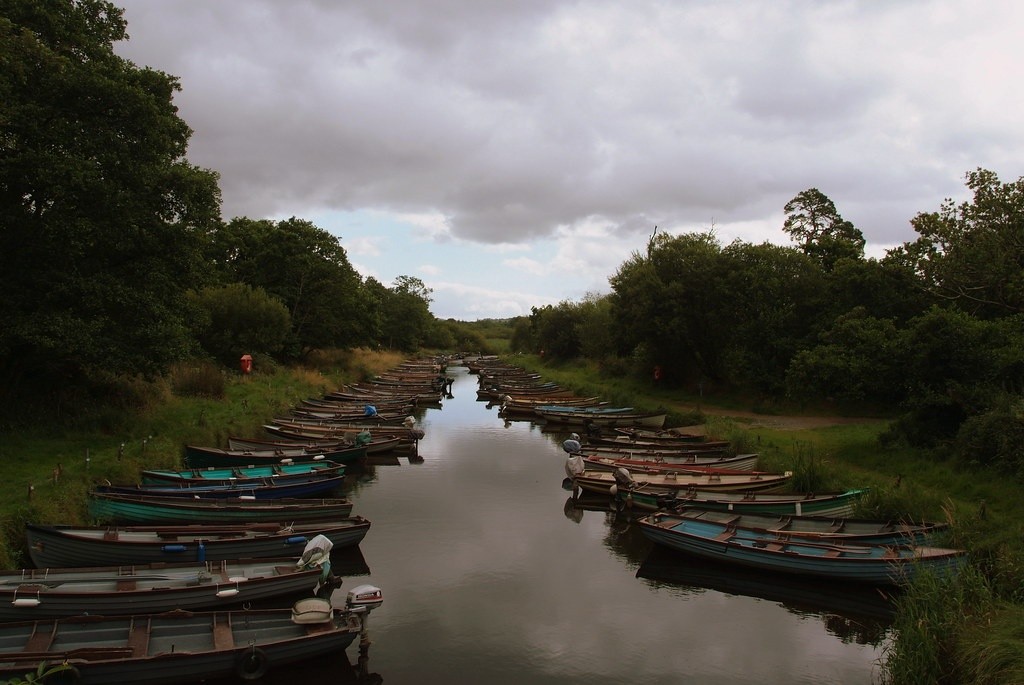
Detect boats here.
[0,533,343,623]
[98,350,794,498]
[655,493,956,546]
[636,510,971,588]
[611,466,868,519]
[87,488,354,525]
[23,516,373,568]
[0,583,388,685]
